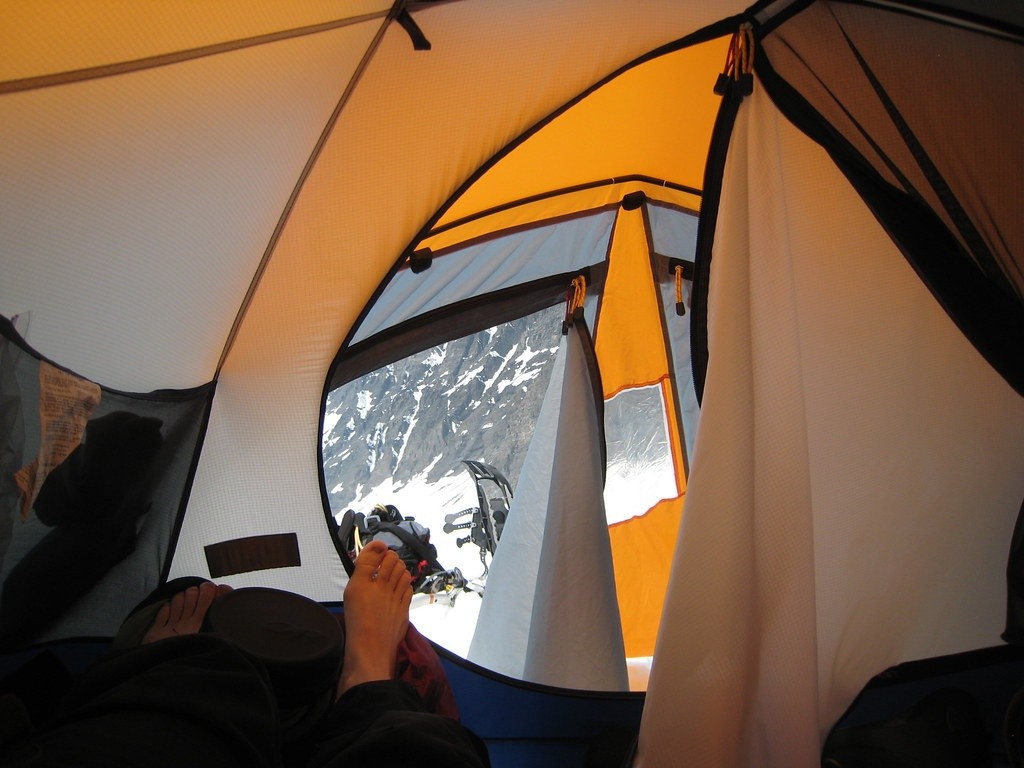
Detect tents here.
[3,1,1024,768]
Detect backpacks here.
[339,504,466,596]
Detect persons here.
[0,541,491,768]
